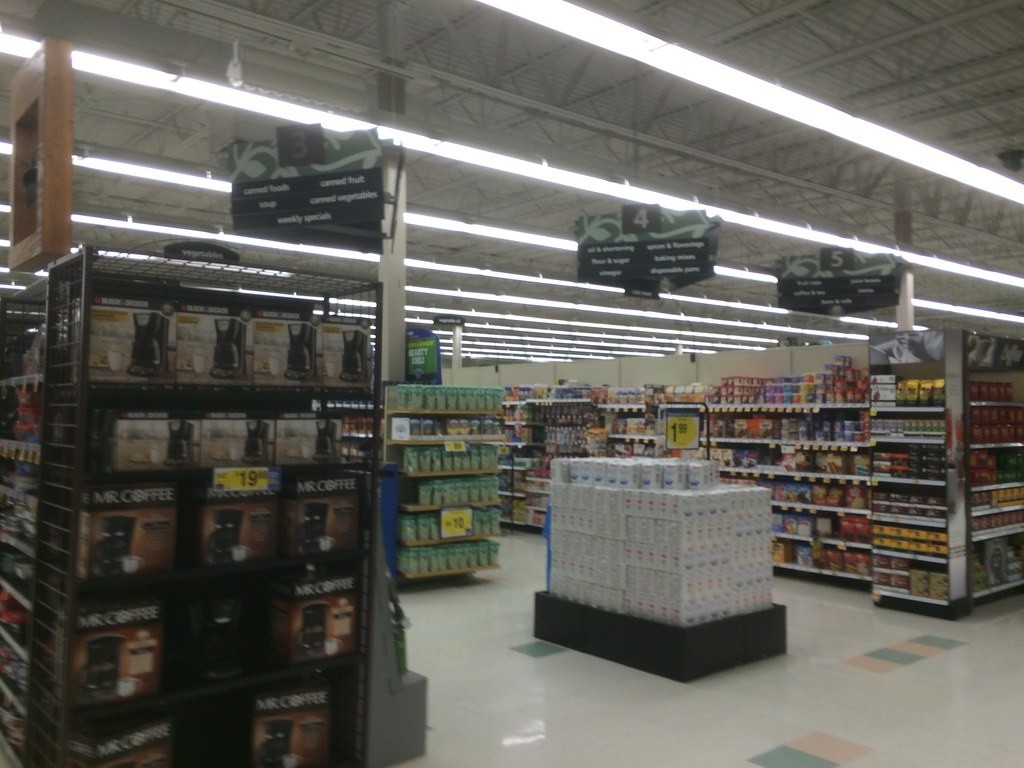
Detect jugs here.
[343,349,362,373]
[316,436,331,453]
[168,438,187,458]
[288,344,310,371]
[296,624,323,651]
[209,530,234,560]
[134,334,160,364]
[80,662,114,690]
[258,740,284,760]
[303,523,321,546]
[99,540,126,572]
[245,438,263,456]
[219,339,239,367]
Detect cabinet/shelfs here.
[653,356,871,585]
[0,262,429,768]
[866,328,1024,618]
[501,392,655,533]
[317,385,501,580]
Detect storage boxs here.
[79,298,360,768]
[702,414,871,578]
[548,456,775,628]
[871,372,1024,602]
[398,384,501,572]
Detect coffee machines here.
[83,311,367,768]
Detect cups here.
[230,448,238,461]
[269,358,281,376]
[327,361,336,377]
[282,754,298,768]
[231,545,251,562]
[319,536,335,551]
[117,678,140,696]
[108,351,124,370]
[194,354,207,373]
[122,555,143,571]
[151,449,161,464]
[302,446,309,457]
[324,637,342,655]
